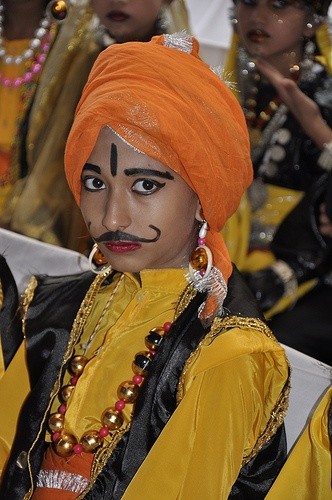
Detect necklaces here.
[0,18,60,89]
[43,270,197,458]
[241,53,301,147]
[0,1,52,66]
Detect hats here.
[64,34,253,319]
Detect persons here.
[220,1,331,193]
[0,34,293,500]
[249,165,331,368]
[6,0,195,257]
[0,1,69,232]
